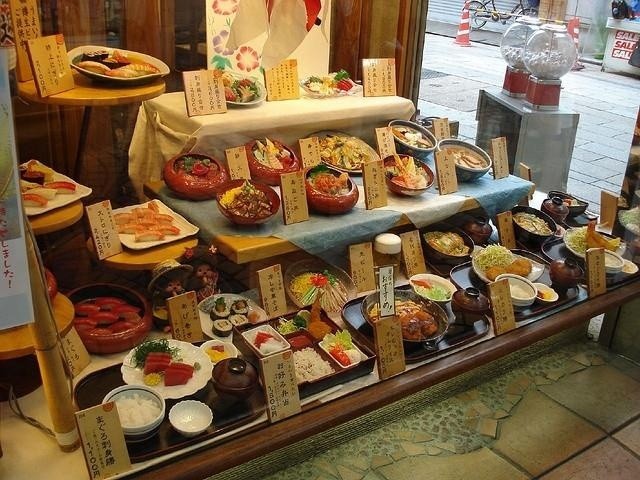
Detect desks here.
[14,51,167,203]
[87,232,200,282]
[24,197,83,243]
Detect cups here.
[374,233,401,286]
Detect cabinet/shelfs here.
[2,73,639,479]
[471,86,579,192]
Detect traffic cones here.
[453,0,473,45]
[565,16,585,71]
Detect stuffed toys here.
[148,244,223,332]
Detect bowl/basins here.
[437,138,493,184]
[408,273,457,305]
[318,336,368,368]
[382,155,434,198]
[462,216,492,244]
[548,257,584,293]
[168,400,214,438]
[299,166,359,214]
[360,288,449,351]
[215,180,281,227]
[420,221,474,265]
[495,272,537,315]
[102,384,165,444]
[451,287,490,328]
[240,323,290,358]
[541,199,570,222]
[585,246,624,279]
[244,139,300,188]
[67,283,152,352]
[162,152,228,201]
[511,205,556,245]
[547,191,587,218]
[199,339,238,366]
[388,120,438,158]
[212,358,259,405]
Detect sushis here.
[211,300,249,337]
[81,50,131,69]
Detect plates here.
[472,248,545,283]
[533,284,559,305]
[67,45,170,87]
[197,293,266,341]
[284,258,358,317]
[554,224,566,239]
[19,158,93,216]
[564,229,587,257]
[223,70,268,106]
[121,340,213,400]
[621,258,639,275]
[109,197,201,252]
[305,130,381,172]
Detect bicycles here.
[461,0,538,30]
